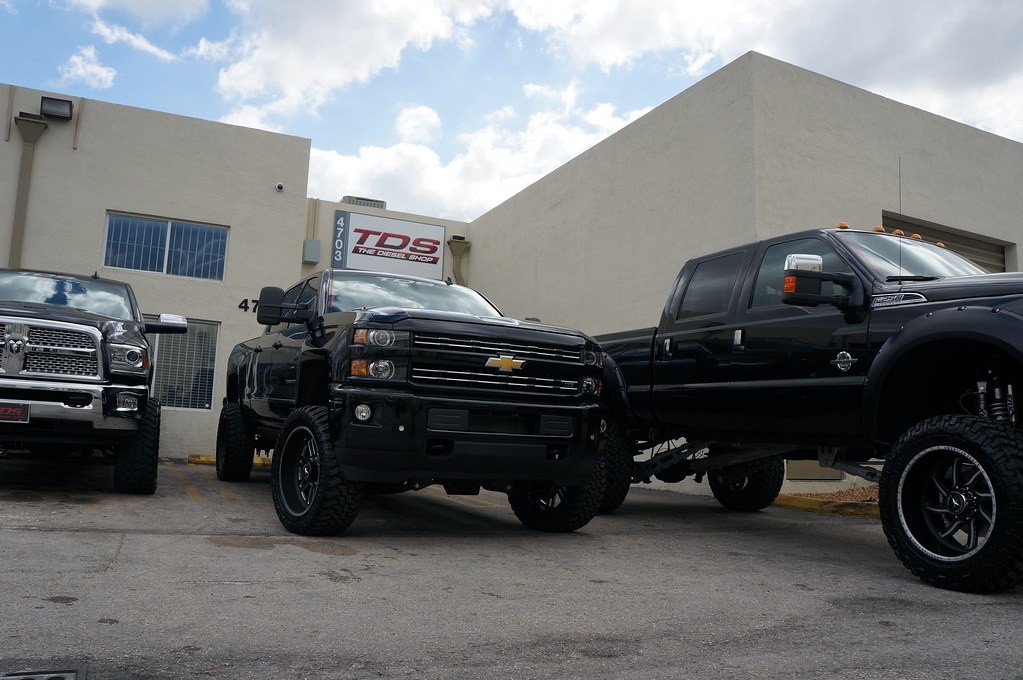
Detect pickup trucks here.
[0,267,189,497]
[588,224,1022,596]
[216,267,607,536]
